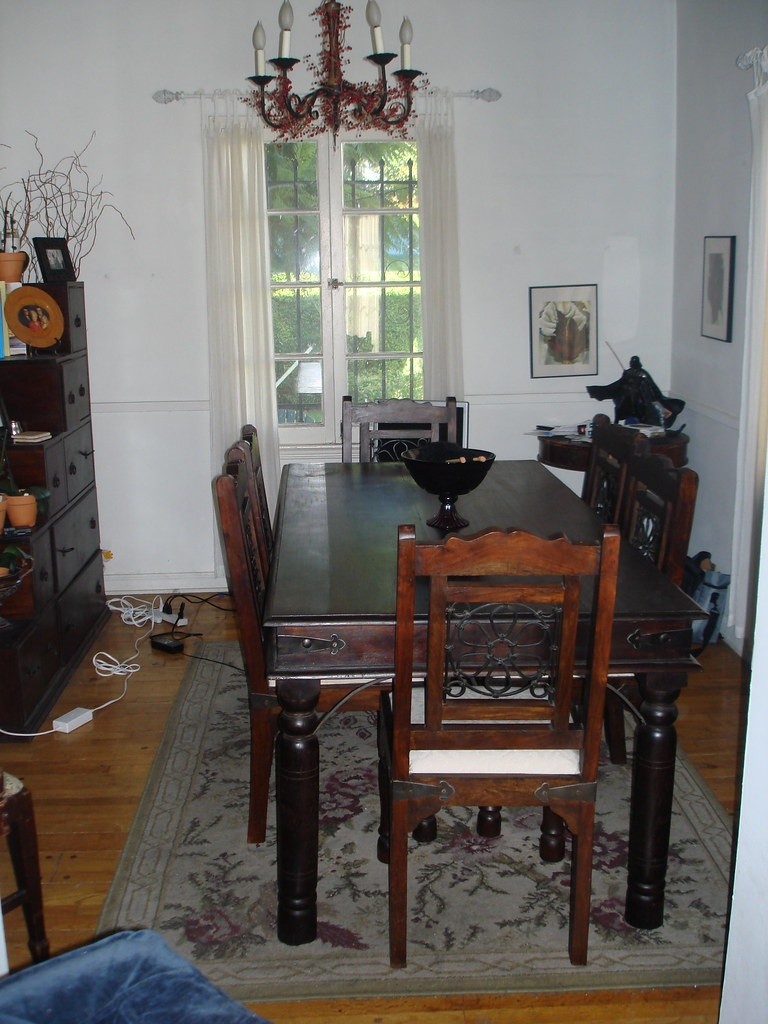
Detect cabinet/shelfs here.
[0,283,112,745]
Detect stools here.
[0,928,270,1024]
[0,772,51,968]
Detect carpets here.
[96,643,734,1007]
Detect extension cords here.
[152,608,188,627]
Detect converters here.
[151,636,184,653]
[53,706,92,733]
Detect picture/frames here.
[33,235,78,284]
[4,286,63,349]
[528,284,599,379]
[701,235,735,342]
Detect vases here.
[0,251,27,283]
[0,493,8,534]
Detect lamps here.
[242,0,431,147]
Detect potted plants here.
[0,474,52,529]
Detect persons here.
[52,251,62,269]
[615,356,661,430]
[22,306,49,331]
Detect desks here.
[537,430,690,473]
[263,461,710,944]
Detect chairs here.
[231,423,275,587]
[377,525,618,968]
[582,414,639,526]
[339,396,457,464]
[214,447,435,845]
[565,433,697,760]
